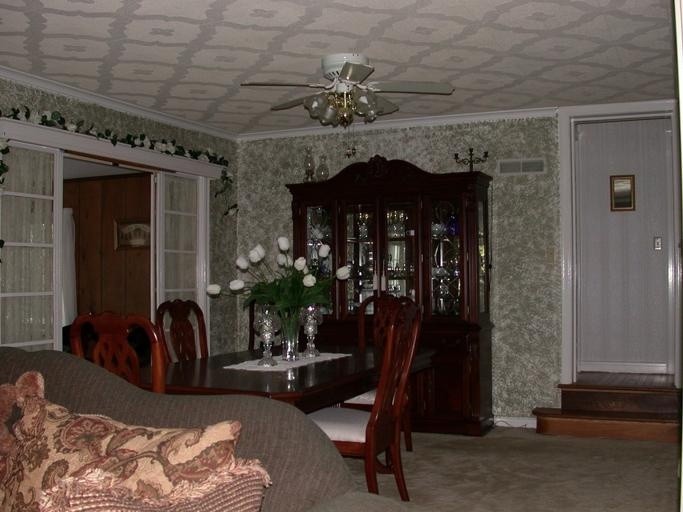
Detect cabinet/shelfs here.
[286,154,494,437]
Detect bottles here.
[356,213,369,241]
[430,205,444,238]
[314,154,329,181]
[445,208,458,234]
[303,153,315,182]
[361,251,373,278]
[387,261,416,277]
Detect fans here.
[240,52,455,110]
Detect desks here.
[121,340,433,416]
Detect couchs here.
[1,347,417,512]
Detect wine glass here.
[392,210,407,239]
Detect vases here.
[280,317,301,362]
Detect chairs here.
[306,297,420,502]
[72,311,164,369]
[156,298,208,363]
[359,294,413,452]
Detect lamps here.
[304,83,378,126]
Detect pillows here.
[1,370,272,511]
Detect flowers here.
[206,237,351,344]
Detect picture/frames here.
[113,219,151,251]
[610,174,635,211]
